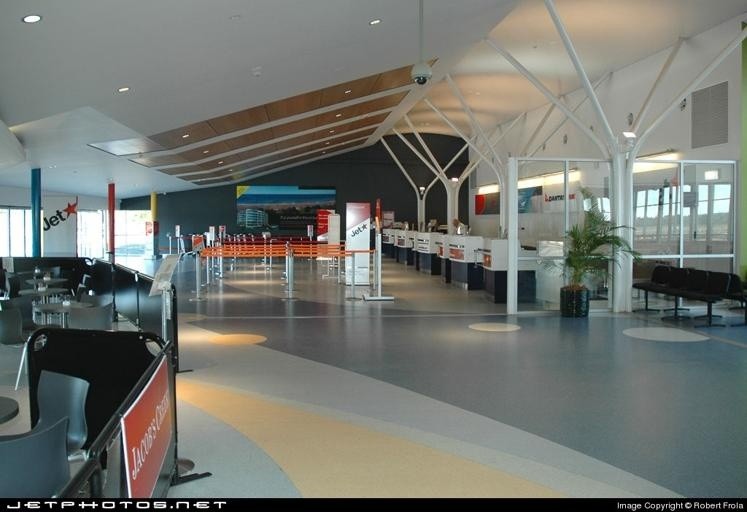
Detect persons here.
[452,219,464,236]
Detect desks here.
[383,229,566,304]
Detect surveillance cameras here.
[410,60,433,86]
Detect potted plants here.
[544,185,641,317]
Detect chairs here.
[0,265,115,498]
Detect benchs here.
[632,265,747,328]
